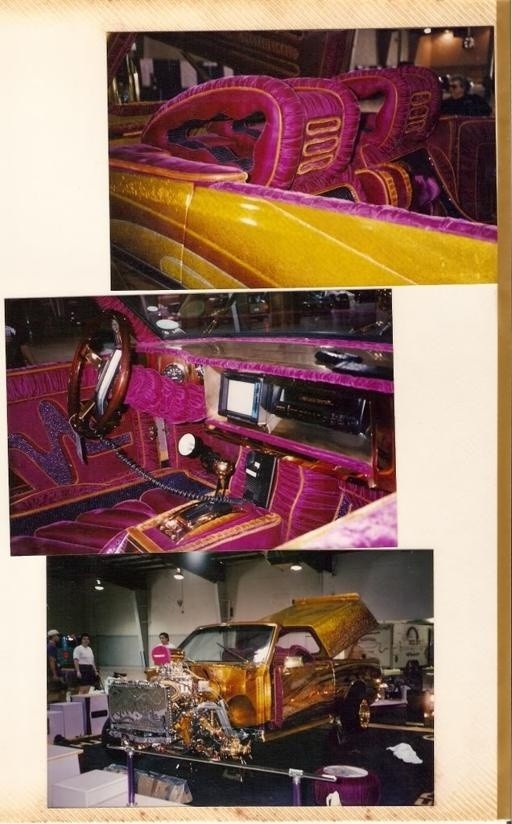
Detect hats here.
[47,629,60,637]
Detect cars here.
[107,32,499,290]
[103,592,383,778]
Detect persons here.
[46,627,68,701]
[155,631,176,649]
[72,632,99,690]
